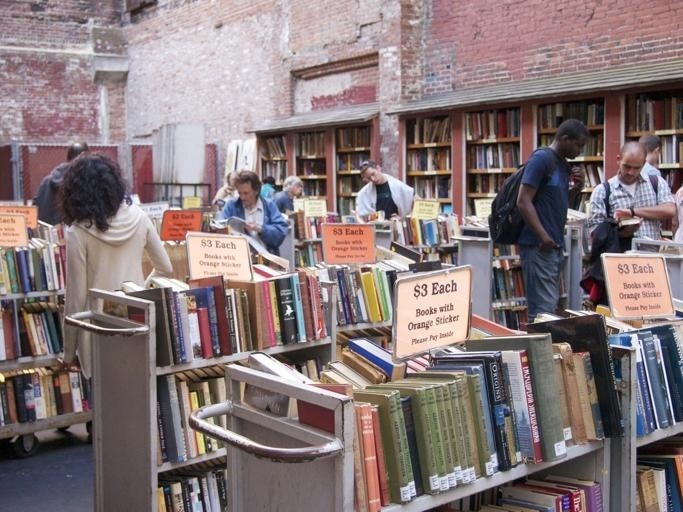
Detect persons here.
[210,168,304,256]
[34,144,91,225]
[516,117,589,324]
[589,133,681,252]
[59,152,173,383]
[355,159,414,220]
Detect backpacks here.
[489,147,552,244]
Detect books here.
[1,220,93,426]
[492,238,565,329]
[101,241,441,511]
[211,217,247,235]
[261,124,370,215]
[304,211,460,265]
[405,101,604,212]
[626,93,681,193]
[242,299,682,512]
